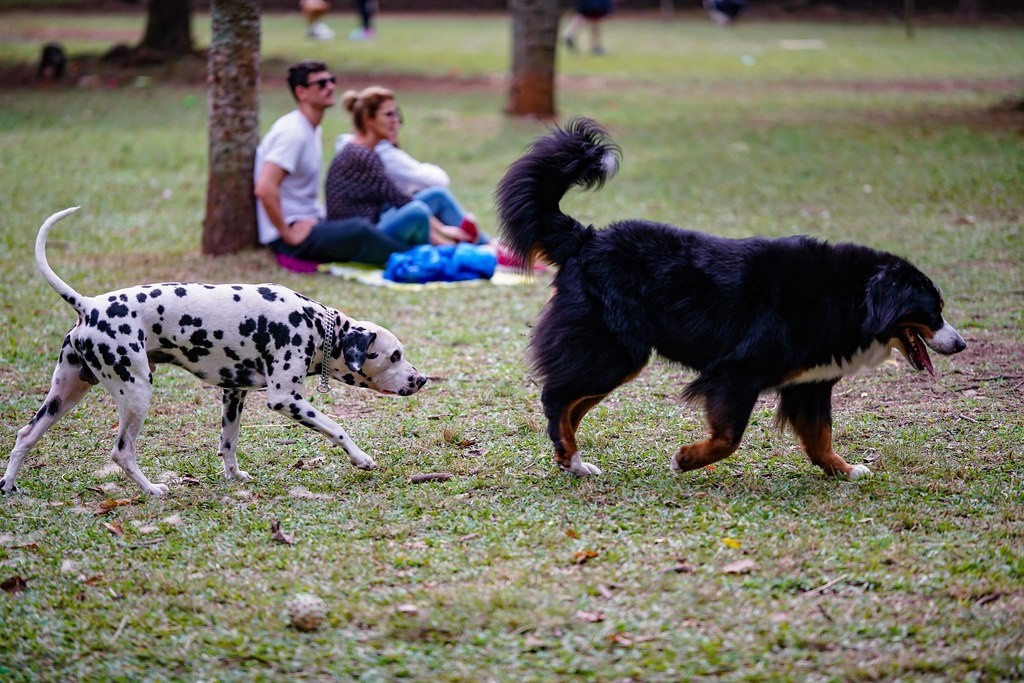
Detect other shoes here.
[494,242,546,273]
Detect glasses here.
[302,75,338,90]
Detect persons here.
[253,61,550,273]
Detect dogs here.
[485,109,966,487]
[0,202,427,496]
